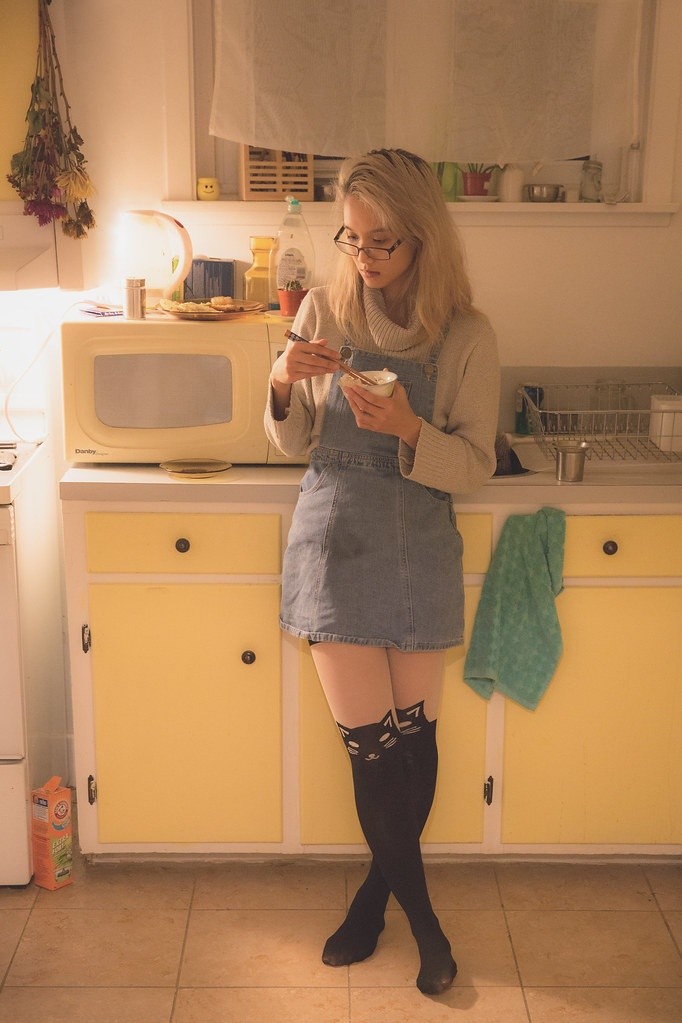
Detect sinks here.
[489,434,538,479]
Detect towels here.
[462,506,567,712]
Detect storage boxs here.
[31,775,73,891]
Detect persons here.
[264,148,501,997]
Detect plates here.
[456,195,499,203]
[159,458,232,479]
[155,298,266,321]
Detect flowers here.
[6,0,98,240]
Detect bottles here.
[124,277,147,320]
[243,236,279,312]
[495,165,526,202]
[268,199,316,310]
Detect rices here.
[342,374,386,386]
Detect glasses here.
[332,225,405,261]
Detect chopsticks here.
[283,328,378,386]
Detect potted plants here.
[456,161,497,195]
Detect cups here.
[550,440,591,483]
[197,177,220,202]
[577,160,603,203]
[516,384,544,434]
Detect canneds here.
[515,383,545,434]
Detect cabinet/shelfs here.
[498,513,681,852]
[293,505,493,847]
[83,507,284,845]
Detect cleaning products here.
[267,195,317,318]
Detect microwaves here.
[60,312,311,464]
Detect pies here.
[159,298,225,313]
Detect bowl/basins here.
[523,183,564,203]
[539,407,579,433]
[338,370,399,398]
